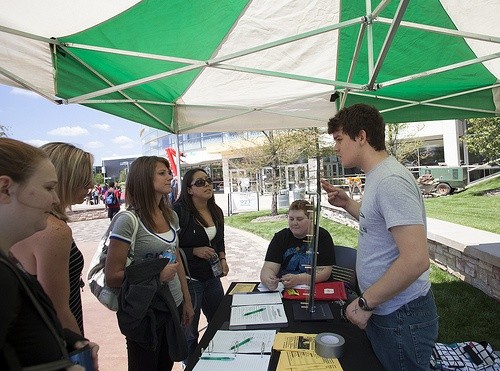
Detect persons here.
[170,166,229,341]
[103,180,120,221]
[106,155,198,371]
[86,183,101,205]
[321,105,438,371]
[0,138,102,371]
[10,142,95,338]
[260,200,336,290]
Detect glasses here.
[190,177,213,187]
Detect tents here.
[0,0,500,210]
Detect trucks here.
[406,165,469,197]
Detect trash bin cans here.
[293,188,305,200]
[278,190,290,209]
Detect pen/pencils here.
[198,357,234,360]
[244,308,266,316]
[231,337,253,350]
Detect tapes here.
[315,333,346,358]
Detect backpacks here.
[105,188,117,206]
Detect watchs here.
[358,292,376,311]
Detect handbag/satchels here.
[87,209,139,312]
[100,195,102,200]
[91,191,95,196]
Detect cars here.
[119,186,126,202]
